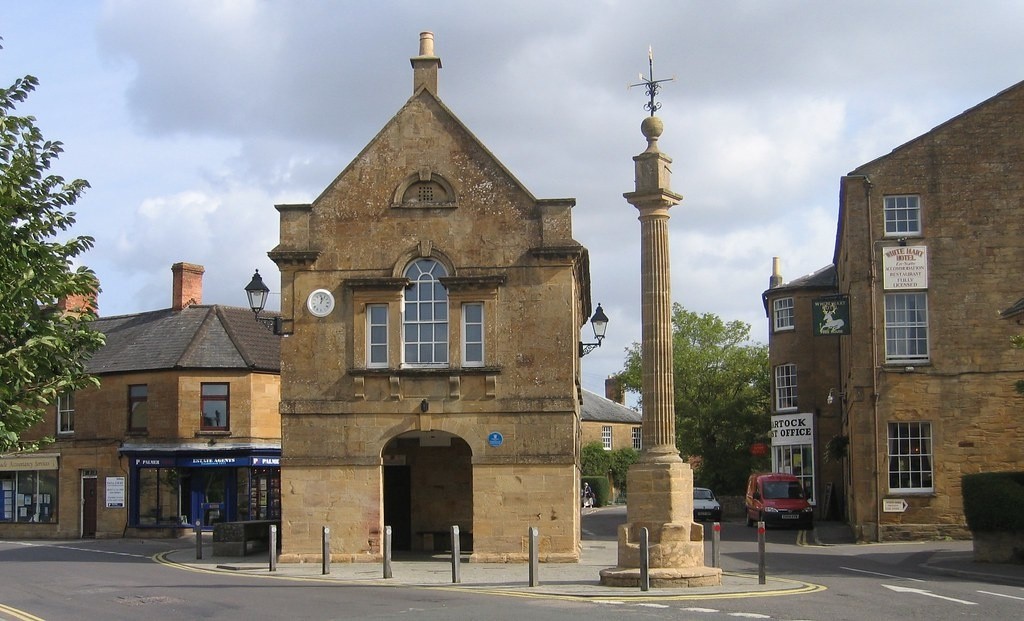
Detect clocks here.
[307,289,335,318]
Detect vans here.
[745,473,812,529]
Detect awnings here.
[0,453,61,470]
[117,443,281,456]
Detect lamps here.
[244,269,275,321]
[579,302,609,358]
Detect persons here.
[582,483,593,508]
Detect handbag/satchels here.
[587,493,591,498]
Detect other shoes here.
[583,506,586,508]
[589,507,592,508]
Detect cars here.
[692,487,720,521]
